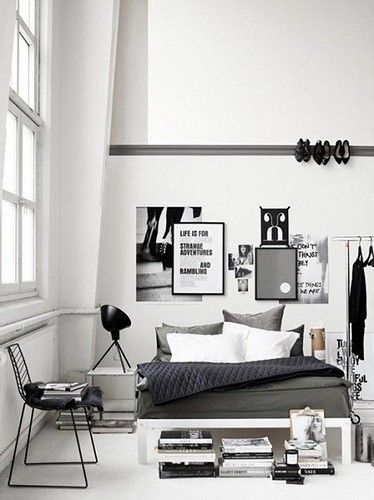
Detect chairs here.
[4,343,99,489]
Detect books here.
[152,430,337,478]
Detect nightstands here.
[86,365,138,414]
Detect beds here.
[134,322,353,466]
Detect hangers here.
[361,236,374,268]
[352,235,365,268]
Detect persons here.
[238,244,253,265]
[305,416,329,458]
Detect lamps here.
[89,303,134,375]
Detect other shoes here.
[314,140,331,165]
[296,139,310,161]
[334,139,350,164]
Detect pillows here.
[223,304,286,333]
[154,321,224,362]
[222,321,301,363]
[164,331,245,365]
